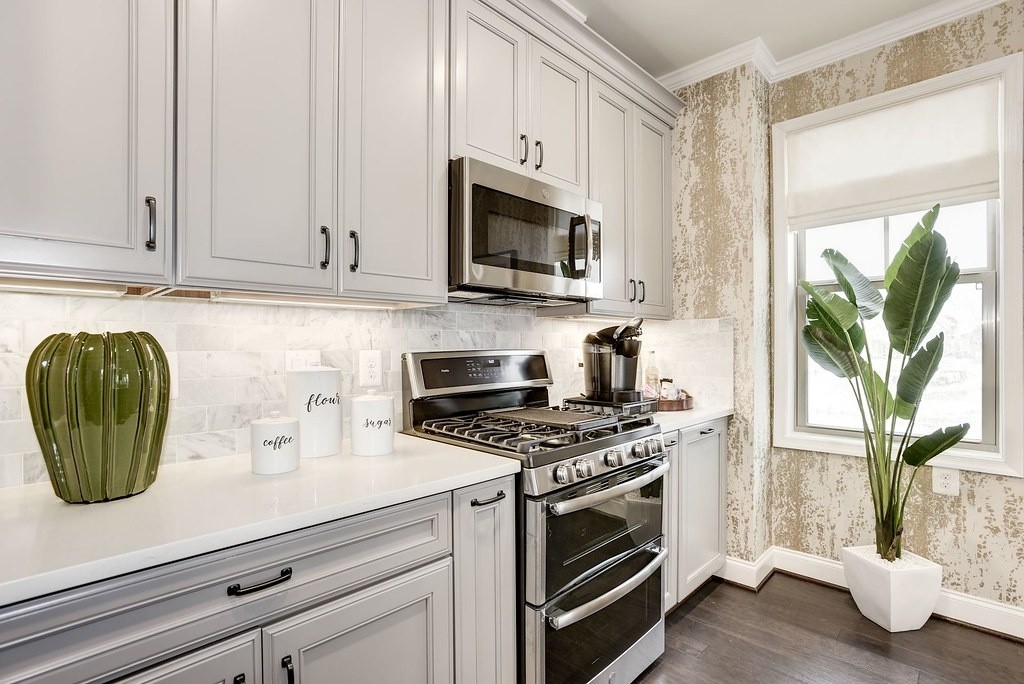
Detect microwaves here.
[449,155,605,310]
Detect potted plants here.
[796,203,969,634]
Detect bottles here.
[645,350,660,398]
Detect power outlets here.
[358,350,383,388]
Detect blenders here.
[581,317,645,403]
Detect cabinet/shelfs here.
[452,474,516,683]
[662,429,678,612]
[449,0,589,197]
[677,416,726,604]
[150,1,450,309]
[0,486,452,683]
[0,0,177,300]
[537,20,676,321]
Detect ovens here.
[527,452,669,684]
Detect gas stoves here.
[399,346,666,498]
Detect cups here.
[350,389,395,456]
[285,360,343,459]
[249,410,300,475]
